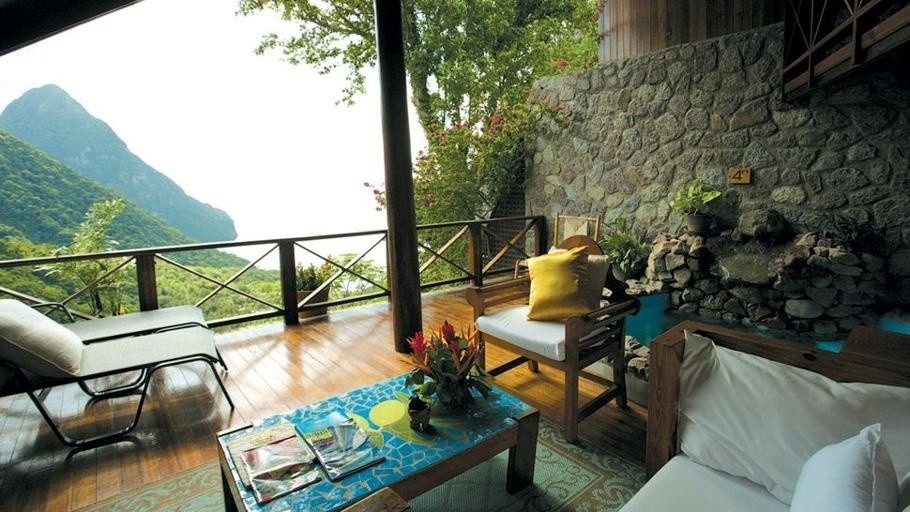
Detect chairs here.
[0,303,235,448]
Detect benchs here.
[614,319,910,512]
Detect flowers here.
[405,320,497,408]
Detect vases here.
[439,390,468,414]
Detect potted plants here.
[597,215,658,285]
[668,179,722,231]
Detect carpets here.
[73,409,650,511]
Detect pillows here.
[0,299,84,380]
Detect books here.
[294,406,385,482]
[241,434,321,506]
[226,420,315,488]
